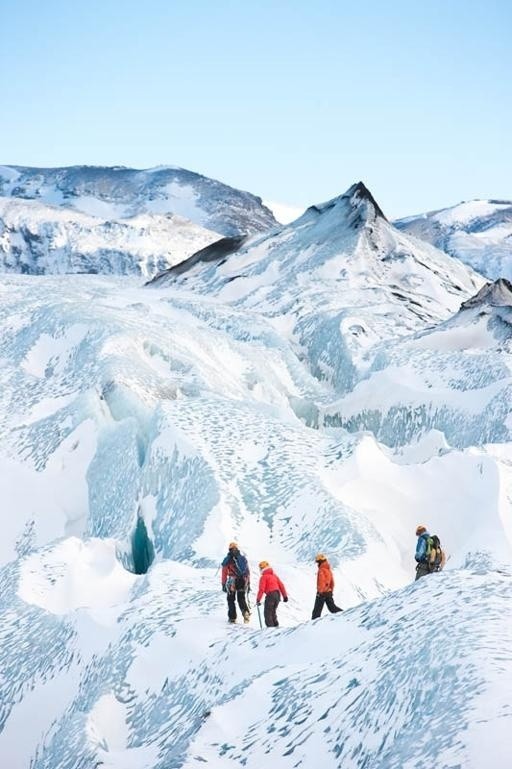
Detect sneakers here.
[228,618,236,624]
[243,611,249,624]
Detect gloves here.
[256,601,262,606]
[223,585,228,593]
[283,596,289,602]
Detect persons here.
[415,528,445,578]
[221,543,344,629]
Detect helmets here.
[315,554,327,561]
[259,561,270,568]
[228,542,238,549]
[415,525,426,535]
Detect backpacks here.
[421,534,446,569]
